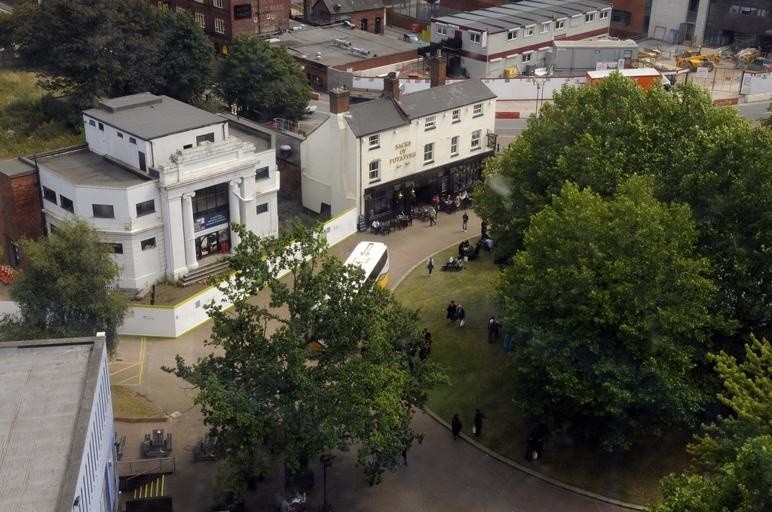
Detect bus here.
[306,242,389,353]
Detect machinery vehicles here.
[675,50,722,72]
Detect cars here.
[748,56,772,72]
[638,46,660,58]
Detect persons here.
[451,412,463,439]
[487,317,498,343]
[503,331,513,367]
[481,218,488,235]
[424,327,432,353]
[473,408,485,435]
[428,206,437,226]
[456,304,465,328]
[428,255,434,275]
[372,219,379,228]
[447,299,456,323]
[432,191,469,215]
[449,235,494,272]
[462,212,470,232]
